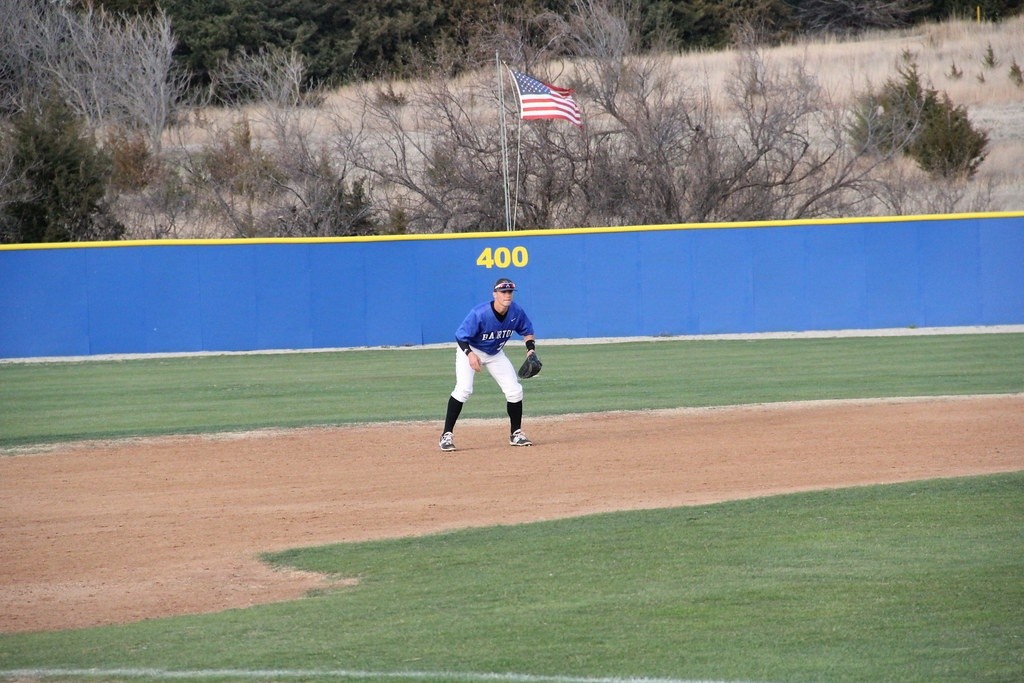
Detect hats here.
[494,279,517,291]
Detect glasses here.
[495,283,515,289]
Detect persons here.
[439,278,542,451]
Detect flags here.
[511,69,582,127]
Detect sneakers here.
[439,431,456,450]
[510,429,532,445]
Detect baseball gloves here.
[517,352,543,379]
